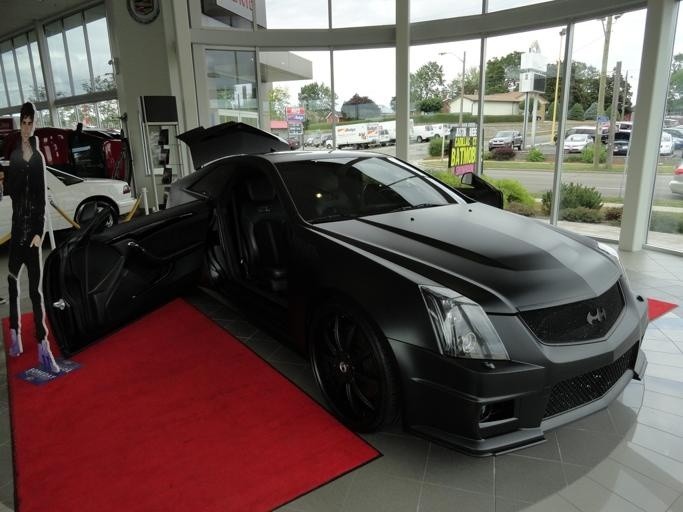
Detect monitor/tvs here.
[140,96,178,126]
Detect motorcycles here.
[304,135,333,148]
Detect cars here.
[0,158,137,247]
[668,163,683,195]
[488,130,522,151]
[0,127,119,176]
[288,138,299,150]
[42,120,648,459]
[414,125,451,143]
[553,115,682,156]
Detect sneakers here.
[10,328,24,353]
[37,338,61,374]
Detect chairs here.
[241,171,353,293]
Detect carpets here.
[2,295,386,511]
[646,295,678,322]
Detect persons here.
[7,101,60,374]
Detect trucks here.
[335,124,391,150]
[380,119,414,146]
